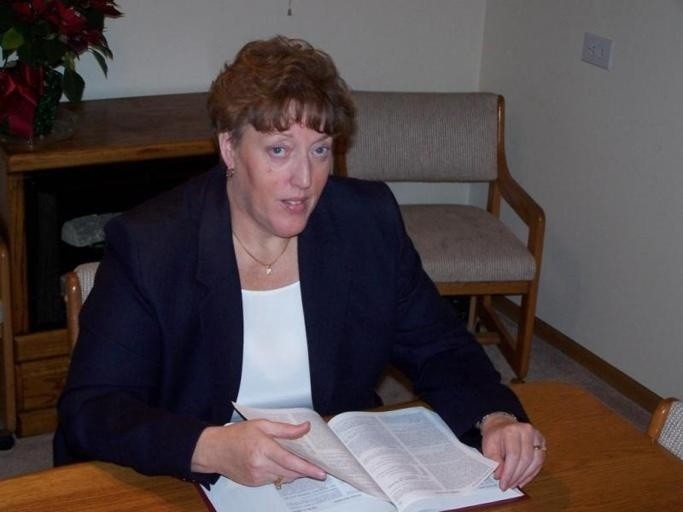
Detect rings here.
[273,474,285,489]
[532,441,547,452]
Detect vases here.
[0,64,64,143]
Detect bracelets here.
[478,410,522,426]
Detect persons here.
[49,33,549,494]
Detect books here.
[193,398,531,511]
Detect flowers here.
[0,0,123,109]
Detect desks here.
[0,382,683,512]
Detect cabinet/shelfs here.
[8,91,216,440]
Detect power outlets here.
[579,32,613,71]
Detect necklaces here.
[231,231,292,277]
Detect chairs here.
[346,88,546,382]
[66,261,99,361]
[649,397,683,462]
